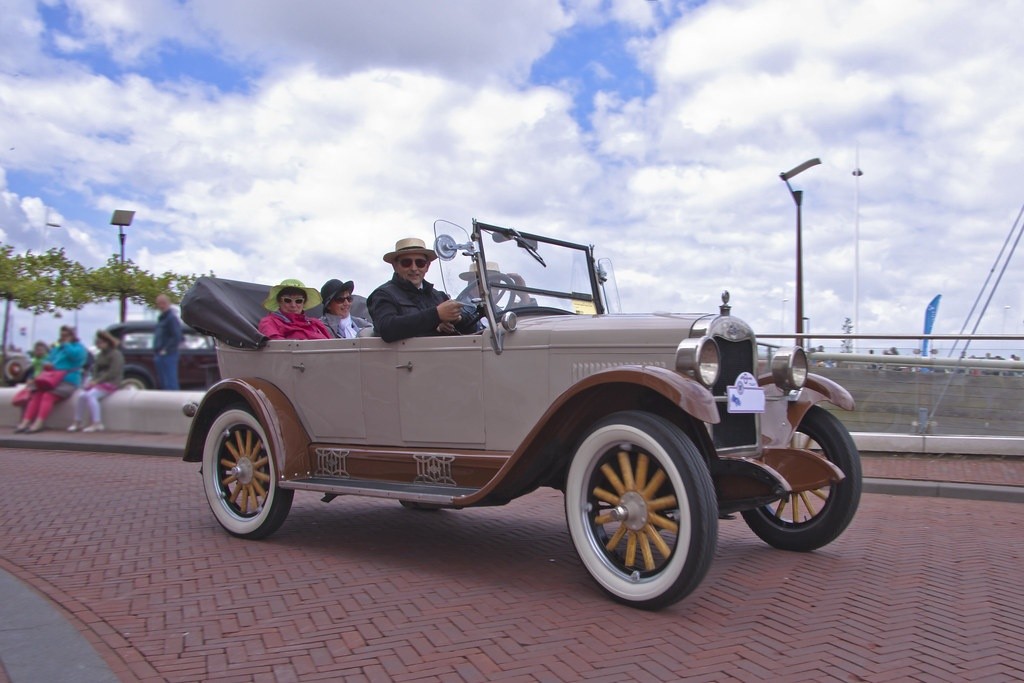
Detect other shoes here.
[66,425,85,432]
[24,425,44,433]
[14,426,29,434]
[82,424,105,432]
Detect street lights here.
[109,211,136,350]
[850,151,865,355]
[802,315,811,350]
[779,158,822,348]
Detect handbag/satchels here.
[35,365,66,390]
[12,387,32,406]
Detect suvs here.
[95,318,220,390]
[180,217,861,611]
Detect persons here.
[318,279,374,339]
[366,238,480,343]
[11,324,125,434]
[152,294,182,390]
[454,262,502,335]
[258,279,336,340]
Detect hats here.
[383,238,437,264]
[63,325,80,342]
[97,330,120,346]
[321,279,354,312]
[263,279,323,312]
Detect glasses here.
[279,296,305,306]
[395,258,430,268]
[333,295,353,304]
